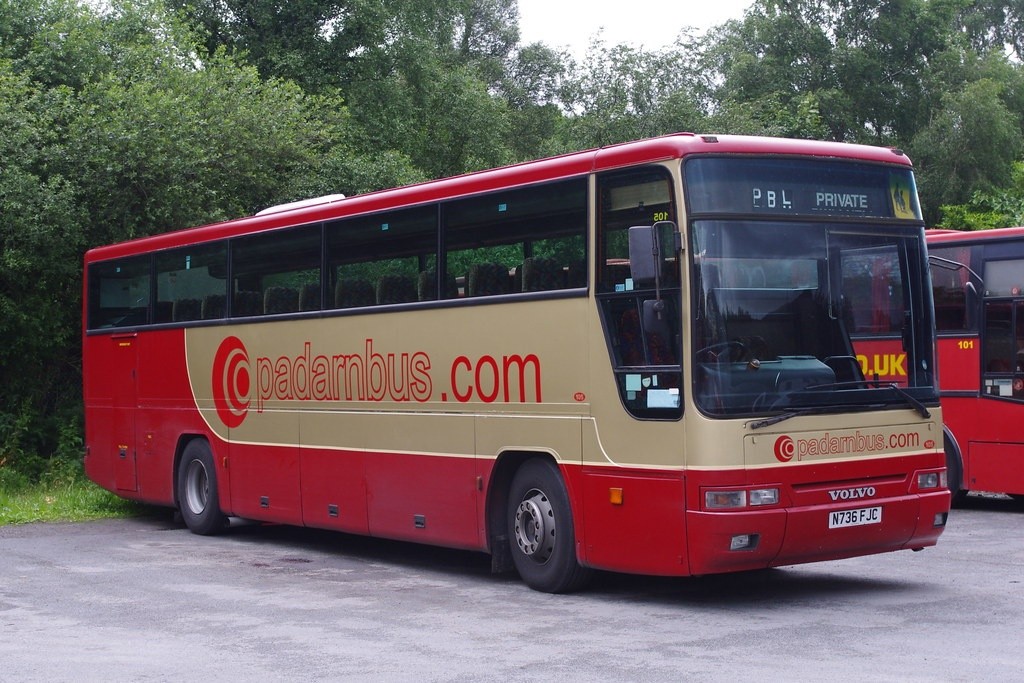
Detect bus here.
[83,131,949,595]
[453,227,1024,507]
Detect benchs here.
[128,260,631,325]
